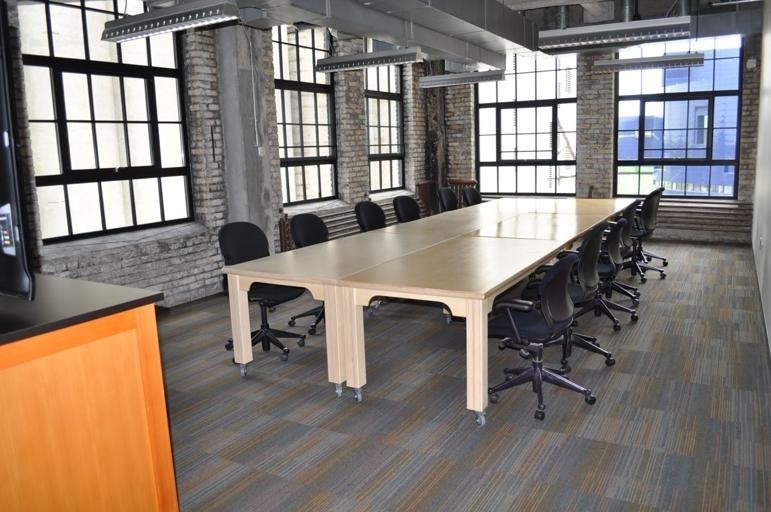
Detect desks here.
[221,231,460,397]
[338,236,568,428]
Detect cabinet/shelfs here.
[0,269,183,512]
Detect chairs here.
[355,187,668,367]
[288,213,329,334]
[218,221,306,364]
[489,254,597,420]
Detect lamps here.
[538,1,691,49]
[314,19,424,73]
[101,1,243,43]
[419,55,505,88]
[594,38,704,72]
